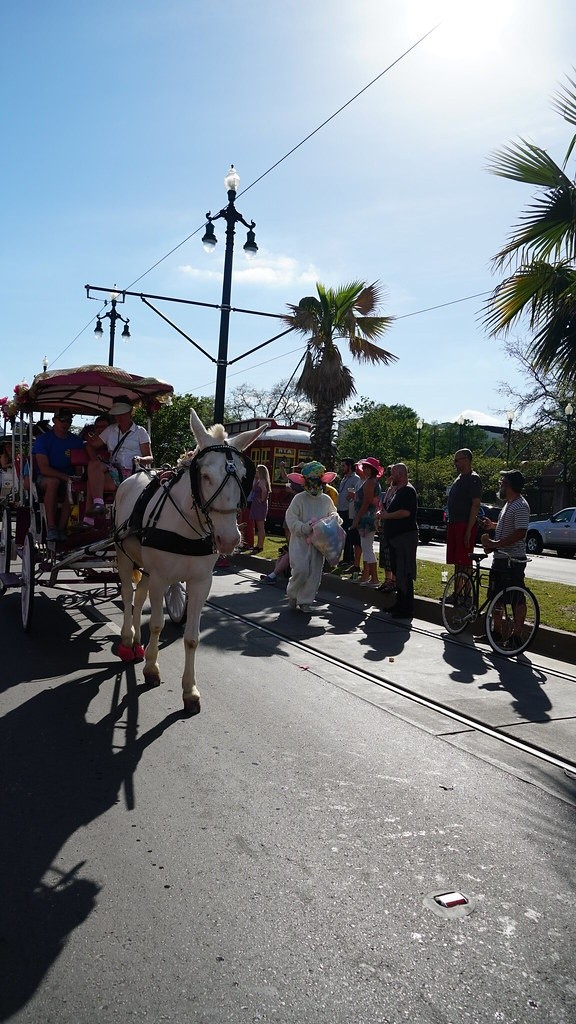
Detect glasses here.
[455,457,468,462]
[57,417,72,423]
[95,425,108,429]
[498,477,508,486]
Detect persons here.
[0,449,31,551]
[250,465,272,553]
[283,463,343,612]
[239,505,255,551]
[260,460,339,585]
[335,459,419,619]
[437,448,482,611]
[473,466,531,649]
[21,393,154,542]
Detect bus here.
[221,418,338,533]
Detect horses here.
[113,409,269,718]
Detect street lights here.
[93,283,133,367]
[506,409,515,468]
[415,419,423,484]
[199,162,258,426]
[561,402,575,509]
[457,414,465,449]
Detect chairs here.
[16,452,116,543]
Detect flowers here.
[0,397,15,422]
[13,385,28,409]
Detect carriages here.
[1,363,270,715]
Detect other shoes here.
[338,560,352,567]
[376,578,397,592]
[299,604,312,612]
[438,591,464,604]
[260,575,277,584]
[500,635,525,650]
[381,604,415,619]
[473,634,504,645]
[360,579,380,586]
[352,578,369,583]
[239,544,254,551]
[289,598,296,608]
[252,546,263,553]
[454,596,472,608]
[47,503,110,542]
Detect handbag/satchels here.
[247,490,254,502]
[343,565,360,574]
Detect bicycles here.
[442,548,540,657]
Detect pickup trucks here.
[416,505,449,543]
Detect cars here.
[524,507,576,554]
[476,502,504,542]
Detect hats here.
[54,407,73,418]
[499,469,525,491]
[362,457,382,474]
[354,458,369,476]
[108,402,132,415]
